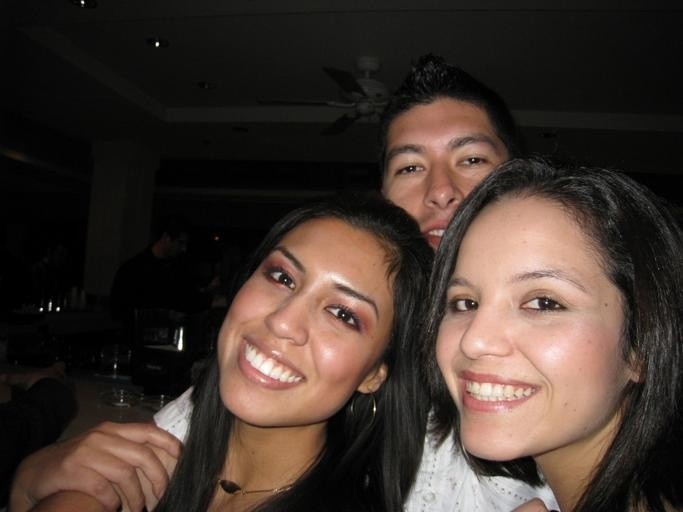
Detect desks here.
[0,366,173,442]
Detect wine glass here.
[100,343,131,391]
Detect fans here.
[254,57,391,137]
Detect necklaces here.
[216,440,292,497]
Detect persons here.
[110,219,221,377]
[362,53,562,511]
[1,359,80,510]
[9,154,682,511]
[27,194,439,511]
